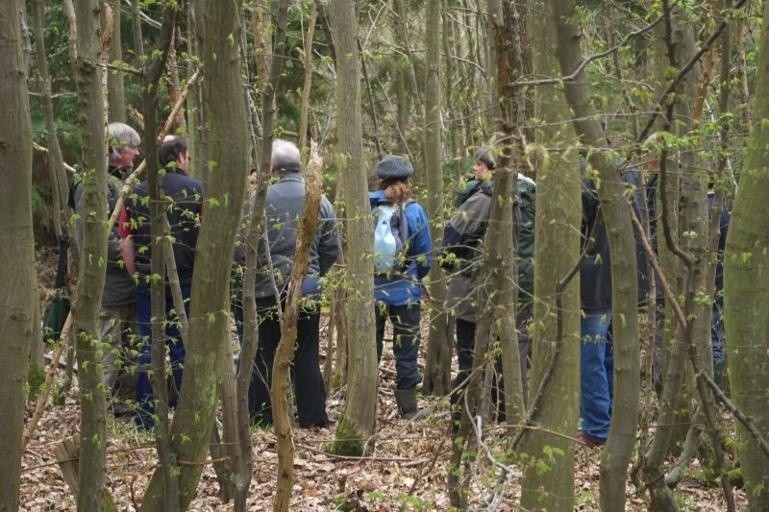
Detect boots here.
[392,388,433,422]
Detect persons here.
[248,169,260,193]
[114,134,205,433]
[67,122,142,418]
[442,131,730,448]
[234,138,341,429]
[368,152,434,420]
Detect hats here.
[474,147,495,169]
[375,154,414,179]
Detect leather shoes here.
[576,429,606,449]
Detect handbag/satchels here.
[42,292,70,344]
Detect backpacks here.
[371,198,416,279]
